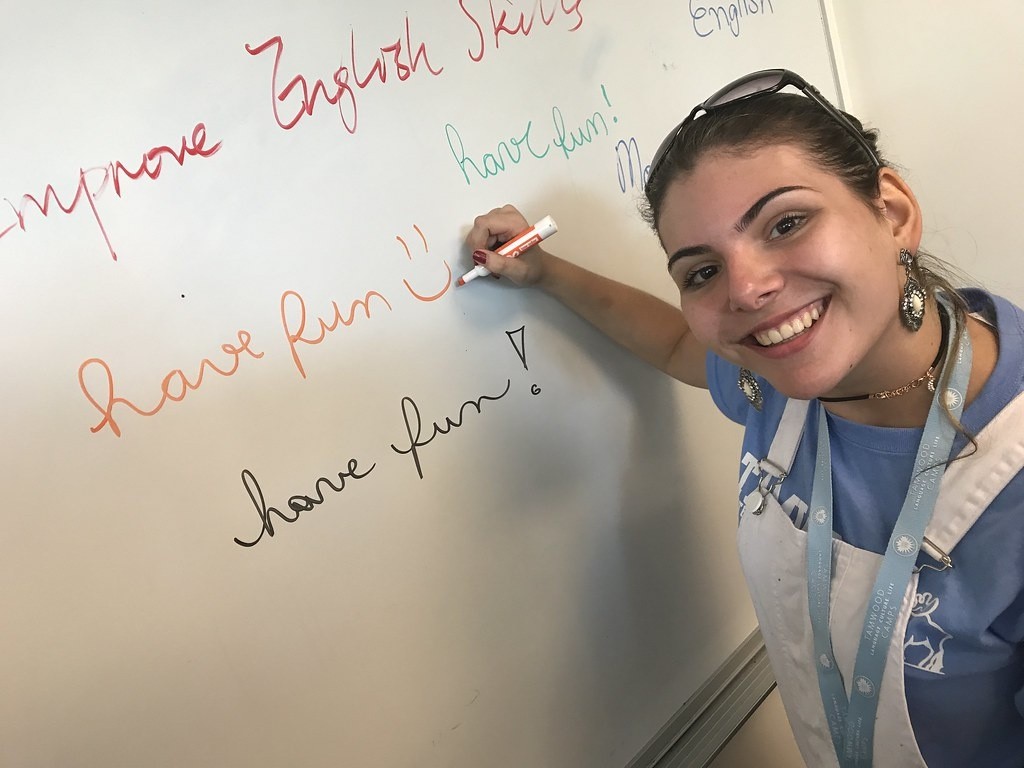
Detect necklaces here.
[817,301,950,402]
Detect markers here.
[458,214,561,287]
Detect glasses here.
[645,69,880,188]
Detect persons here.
[466,68,1024,768]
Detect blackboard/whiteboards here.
[0,0,851,768]
